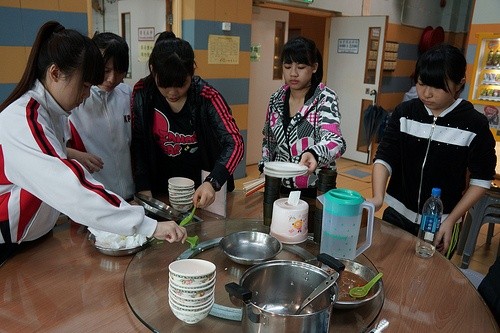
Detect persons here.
[365,45,497,260]
[129,30,245,208]
[67,30,133,202]
[0,20,188,267]
[257,35,347,201]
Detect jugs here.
[317,188,375,260]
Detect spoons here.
[187,236,199,248]
[349,272,383,298]
[180,206,198,226]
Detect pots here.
[224,253,345,333]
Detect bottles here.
[485,50,500,70]
[414,187,443,256]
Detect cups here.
[263,175,281,225]
[314,169,337,241]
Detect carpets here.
[336,166,372,183]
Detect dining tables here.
[0,191,500,333]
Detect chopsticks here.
[242,178,265,197]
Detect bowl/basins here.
[169,258,217,325]
[168,177,195,213]
[311,259,381,308]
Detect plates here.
[218,229,283,265]
[262,162,308,178]
[134,192,178,222]
[89,234,149,255]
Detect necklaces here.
[170,98,184,111]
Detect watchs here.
[203,176,221,192]
